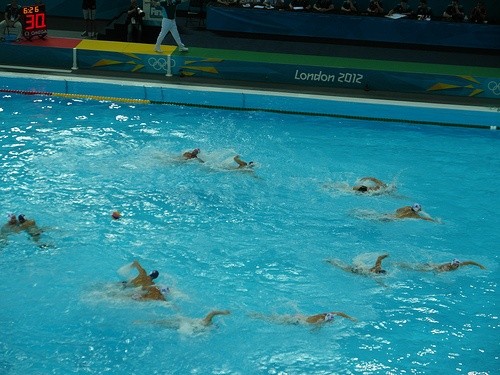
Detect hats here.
[451,259,460,268]
[18,214,27,222]
[412,204,421,211]
[149,270,159,279]
[324,313,334,321]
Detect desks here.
[206,3,500,53]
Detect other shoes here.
[80,32,89,37]
[154,48,163,52]
[0,37,5,40]
[179,46,189,52]
[89,33,96,37]
[16,38,23,41]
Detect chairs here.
[1,25,17,44]
[185,0,204,28]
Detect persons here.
[203,311,230,325]
[152,0,189,53]
[184,148,253,169]
[217,0,290,12]
[340,0,359,13]
[4,211,36,233]
[124,260,170,302]
[443,0,465,18]
[103,0,137,26]
[388,0,434,20]
[306,254,488,323]
[0,0,23,42]
[366,0,384,13]
[112,211,121,221]
[313,0,335,12]
[81,0,96,37]
[289,0,312,11]
[468,0,488,24]
[396,202,438,221]
[352,177,385,193]
